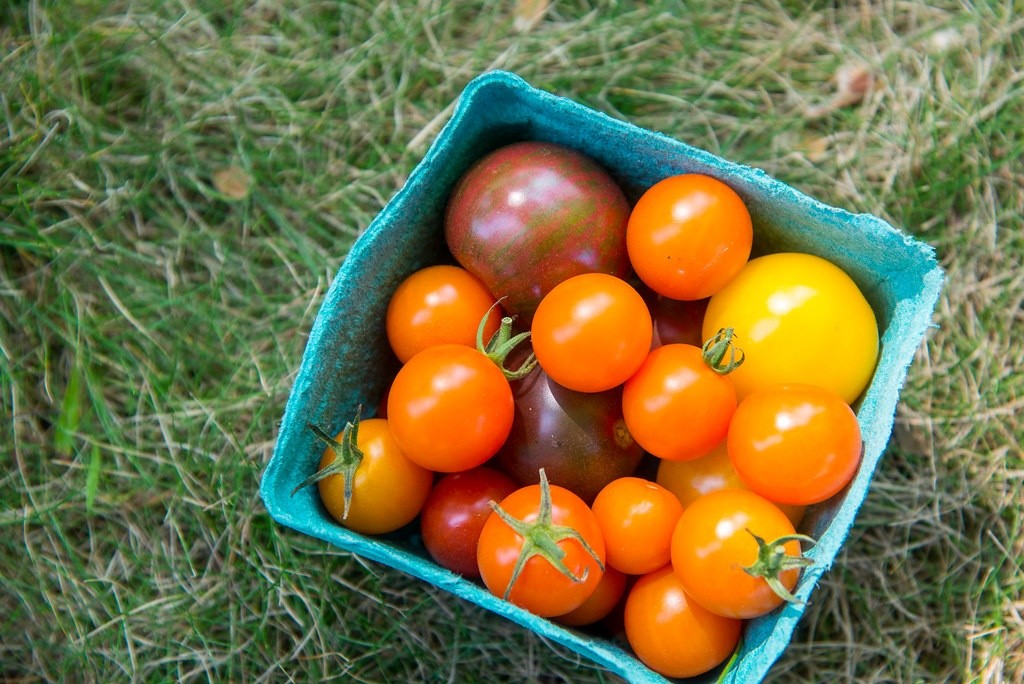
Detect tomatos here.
[290,140,879,679]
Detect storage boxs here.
[261,70,943,683]
